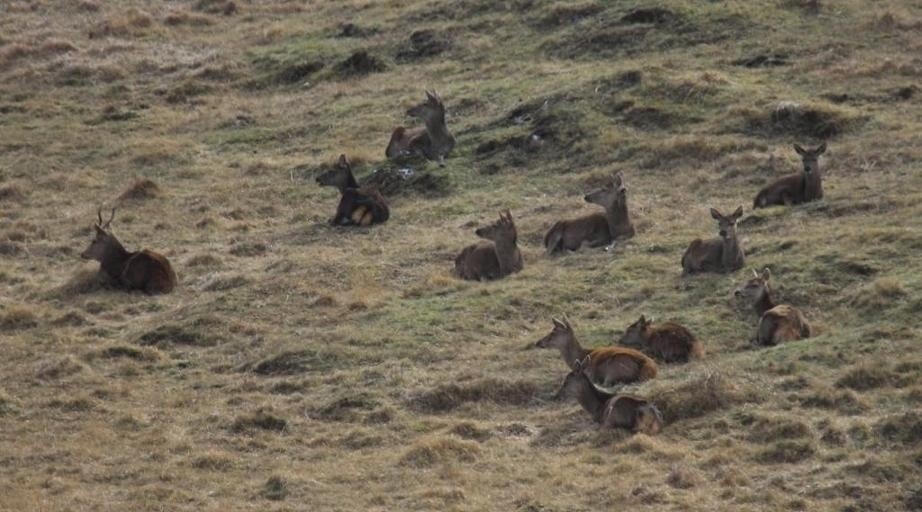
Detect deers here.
[617,314,705,365]
[314,153,390,227]
[385,89,455,167]
[752,142,828,211]
[681,205,744,276]
[79,204,176,295]
[734,265,811,346]
[544,170,636,257]
[553,354,664,436]
[455,208,524,283]
[535,311,657,387]
